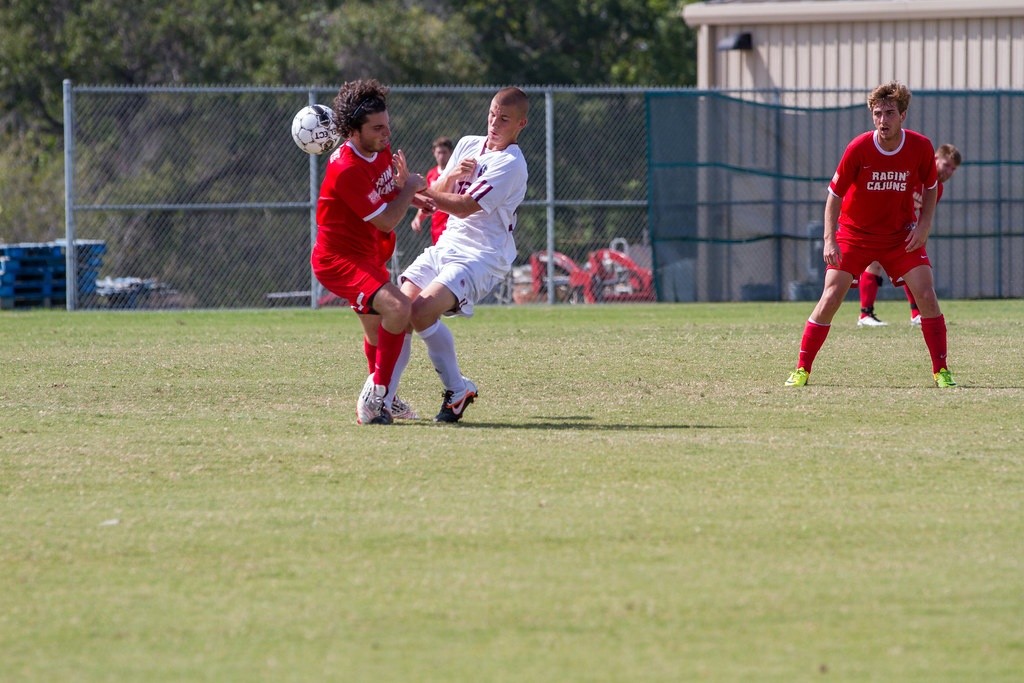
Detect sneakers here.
[372,408,391,425]
[858,313,887,326]
[911,314,922,324]
[932,367,957,388]
[784,366,810,387]
[356,372,389,424]
[433,376,478,423]
[383,393,410,418]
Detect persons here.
[856,144,961,326]
[411,136,454,246]
[311,79,412,424]
[784,83,956,389]
[372,88,531,425]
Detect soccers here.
[291,105,341,154]
